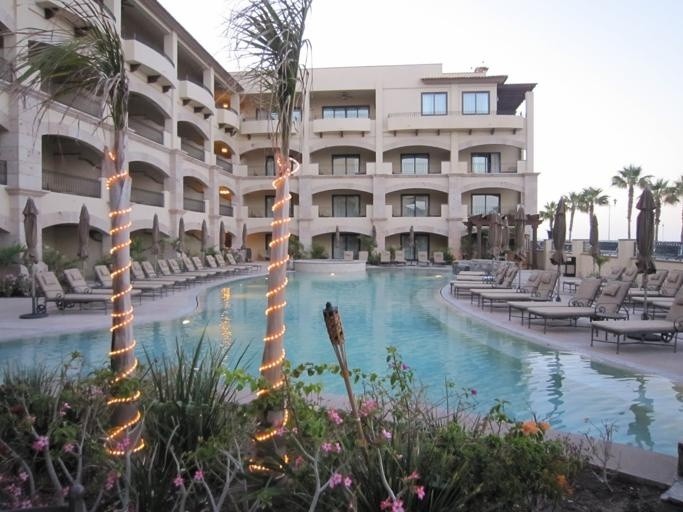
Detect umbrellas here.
[75,203,91,277]
[407,225,415,264]
[21,198,39,316]
[588,213,602,261]
[176,217,187,257]
[546,195,570,272]
[635,187,659,321]
[486,208,503,262]
[240,222,247,249]
[198,219,206,268]
[501,215,510,263]
[219,219,225,259]
[334,224,339,258]
[151,213,160,276]
[511,204,527,271]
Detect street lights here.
[606,198,616,241]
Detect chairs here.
[32,252,260,316]
[449,259,682,355]
[338,250,448,266]
